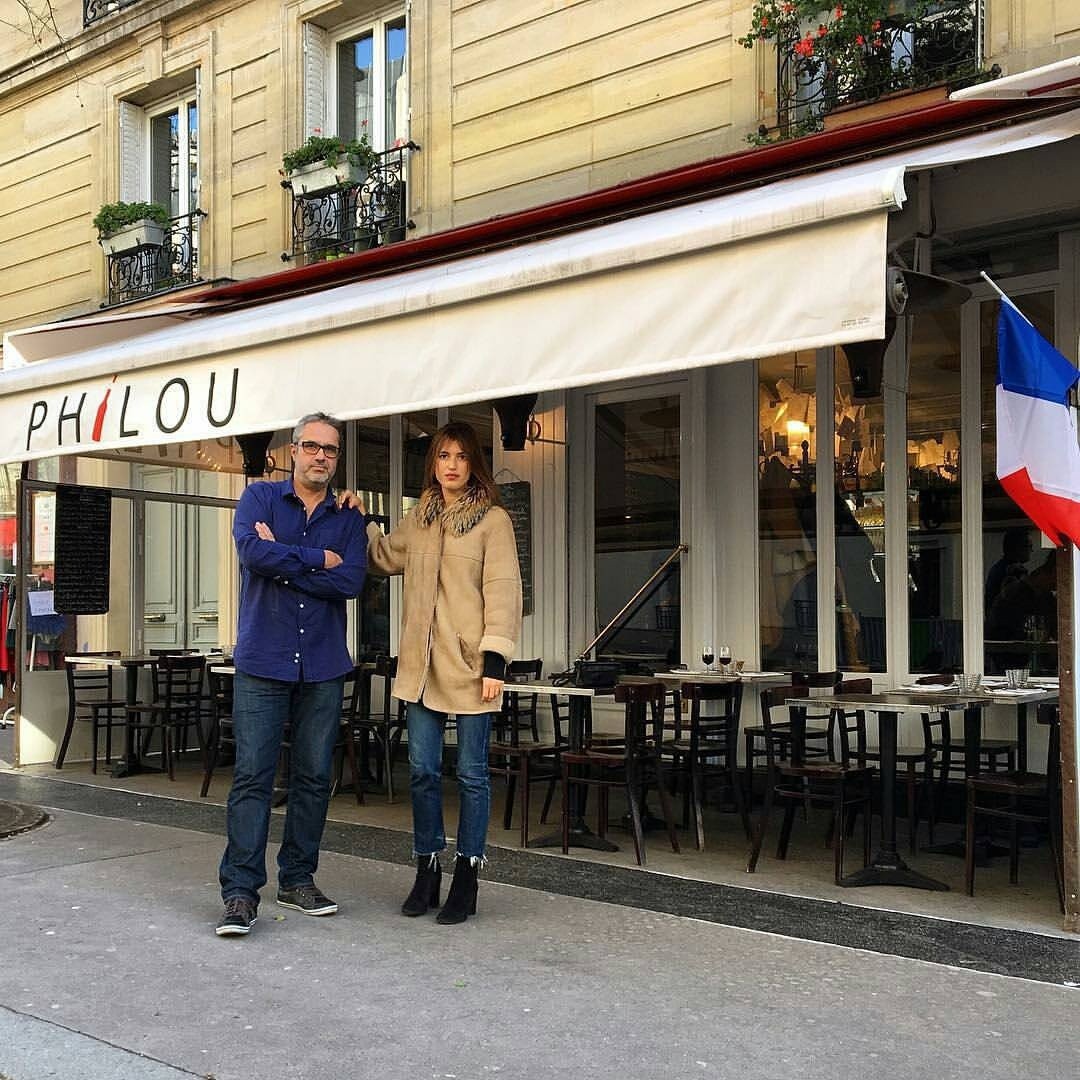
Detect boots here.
[435,851,485,925]
[401,851,442,917]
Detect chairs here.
[55,649,1065,914]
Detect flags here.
[991,293,1080,552]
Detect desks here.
[505,675,683,852]
[783,693,994,892]
[654,671,791,814]
[338,663,396,796]
[880,682,1060,867]
[984,640,1059,677]
[210,667,290,807]
[65,656,195,780]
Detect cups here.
[222,645,233,658]
[182,650,191,656]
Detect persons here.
[977,526,1033,674]
[334,420,522,924]
[213,411,369,936]
[1013,549,1059,643]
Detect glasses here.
[294,441,343,458]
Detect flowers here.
[735,0,975,147]
[278,120,383,192]
[338,244,349,254]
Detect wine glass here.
[719,646,731,675]
[701,646,714,675]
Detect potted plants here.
[353,227,405,254]
[93,201,172,259]
[326,250,337,261]
[310,237,348,263]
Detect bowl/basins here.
[1006,668,1030,686]
[953,673,981,692]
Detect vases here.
[291,152,367,199]
[824,79,950,130]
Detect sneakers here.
[214,897,258,935]
[276,884,338,915]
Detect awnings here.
[0,173,901,460]
